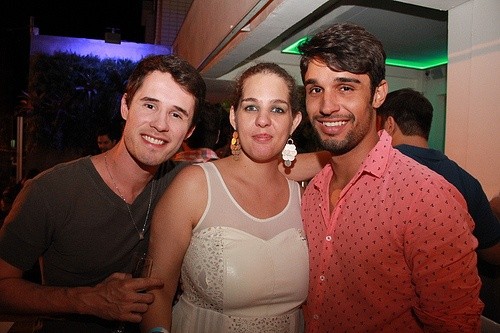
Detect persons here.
[377,87,500,265]
[301,21,500,333]
[96,127,117,152]
[170,101,223,163]
[140,62,309,333]
[0,54,330,333]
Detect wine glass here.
[110,251,152,333]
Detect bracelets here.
[146,327,169,333]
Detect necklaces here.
[104,150,154,239]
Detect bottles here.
[11,136,16,148]
